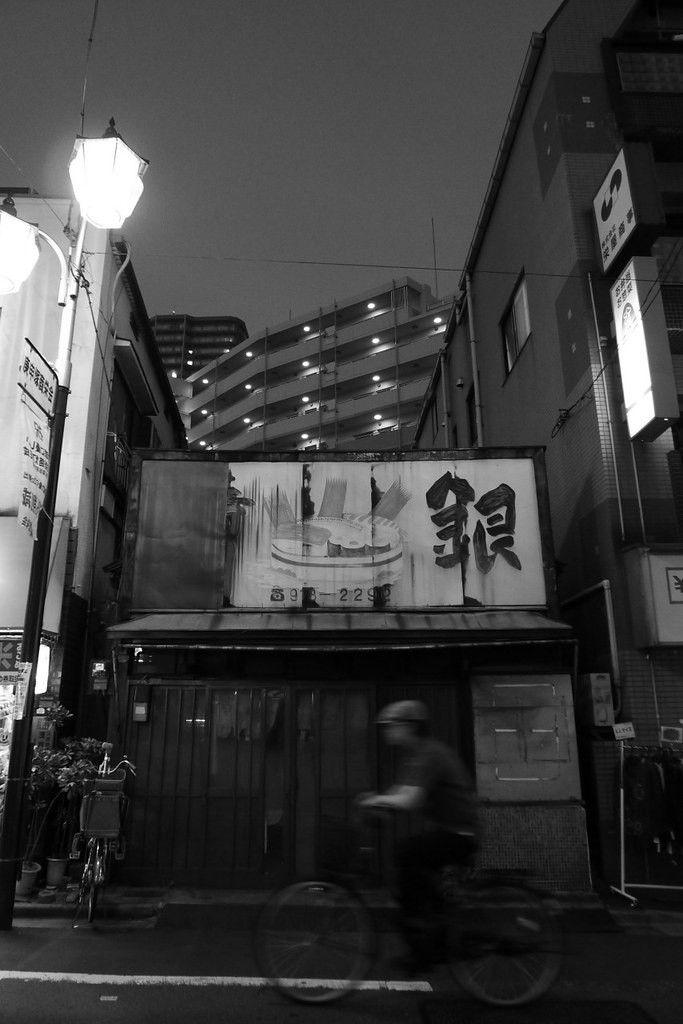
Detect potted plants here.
[15,699,109,896]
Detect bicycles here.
[253,846,560,1008]
[75,755,136,923]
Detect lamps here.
[456,379,464,390]
[137,652,151,665]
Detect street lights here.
[0,116,150,930]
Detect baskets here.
[82,769,127,796]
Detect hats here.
[372,701,430,725]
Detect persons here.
[359,699,480,971]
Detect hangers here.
[626,745,681,765]
